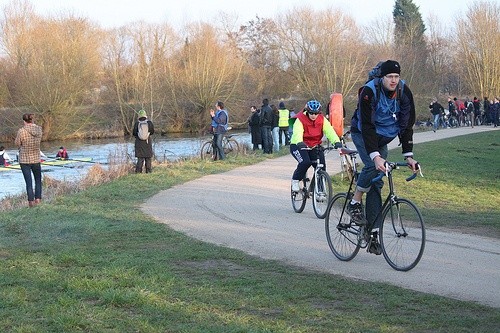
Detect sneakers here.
[346,200,367,225]
[367,232,382,255]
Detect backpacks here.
[138,121,149,140]
[365,62,402,105]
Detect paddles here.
[39,150,110,165]
[39,161,84,169]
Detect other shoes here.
[292,180,300,192]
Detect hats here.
[279,102,285,108]
[138,110,146,119]
[380,60,401,77]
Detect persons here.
[260,99,274,154]
[288,107,296,142]
[270,104,279,152]
[0,146,14,167]
[16,147,20,162]
[415,119,424,127]
[454,97,492,126]
[132,110,154,173]
[345,60,419,255]
[40,150,47,162]
[14,113,43,207]
[490,97,500,128]
[429,97,444,133]
[289,100,343,202]
[447,97,456,127]
[56,146,69,161]
[249,106,261,150]
[426,118,433,126]
[209,100,228,161]
[278,101,291,148]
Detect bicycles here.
[338,130,361,185]
[290,144,337,220]
[201,135,240,160]
[444,112,487,129]
[324,150,427,272]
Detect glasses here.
[309,112,318,115]
[386,75,400,79]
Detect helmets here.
[307,100,320,113]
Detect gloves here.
[334,142,342,149]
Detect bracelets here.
[212,114,215,117]
